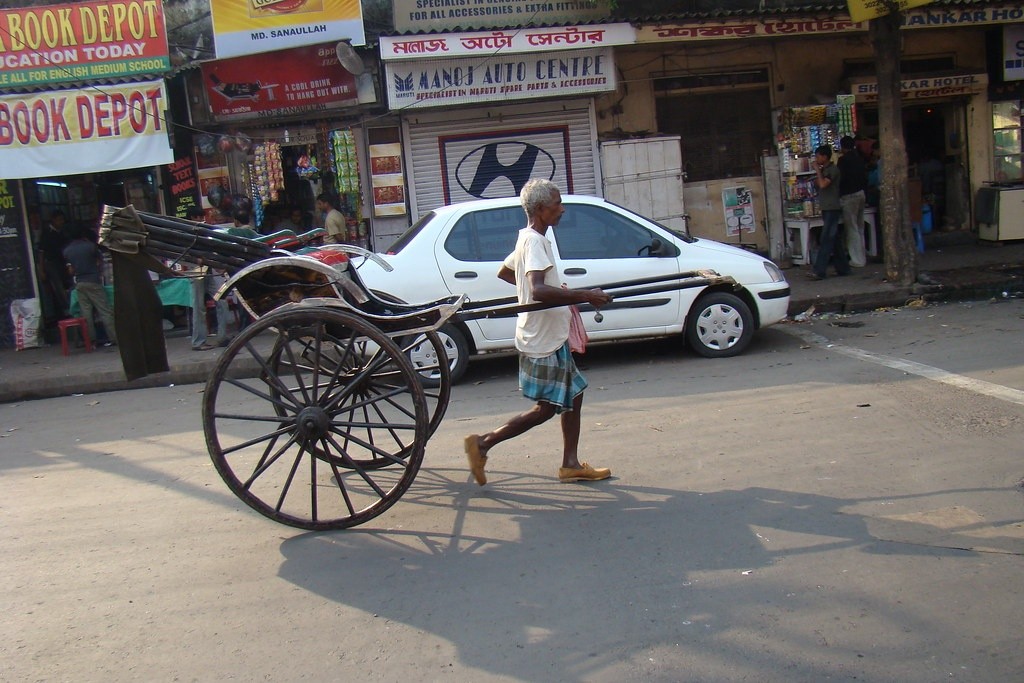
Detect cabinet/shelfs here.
[979,183,1024,241]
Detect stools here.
[58,317,93,356]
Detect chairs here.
[207,225,468,353]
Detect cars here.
[337,196,791,386]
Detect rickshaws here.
[96,203,729,530]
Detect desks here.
[71,274,194,350]
[784,206,878,266]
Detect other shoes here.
[193,343,216,350]
[830,271,840,276]
[559,462,611,483]
[219,340,230,347]
[464,434,488,486]
[804,272,823,280]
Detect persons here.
[804,145,854,280]
[837,135,867,269]
[867,140,885,263]
[29,211,118,349]
[465,178,611,488]
[228,207,259,334]
[317,192,348,243]
[936,129,968,180]
[272,205,306,234]
[183,206,234,351]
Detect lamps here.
[335,41,370,76]
